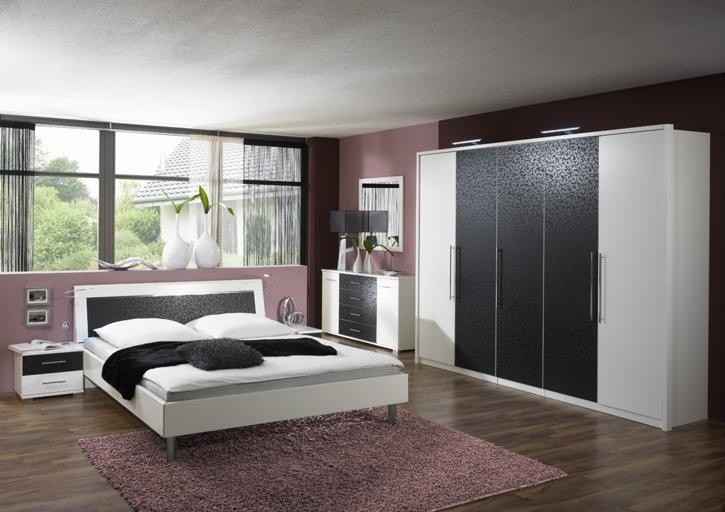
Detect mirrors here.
[357,175,405,254]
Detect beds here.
[72,278,410,463]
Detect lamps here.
[329,209,363,272]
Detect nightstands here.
[7,338,85,400]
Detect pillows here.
[91,311,299,373]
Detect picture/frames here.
[23,286,52,329]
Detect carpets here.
[76,405,570,512]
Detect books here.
[8,339,64,352]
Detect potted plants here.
[338,235,401,274]
[159,184,235,270]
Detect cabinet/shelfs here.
[412,123,710,433]
[320,269,415,354]
